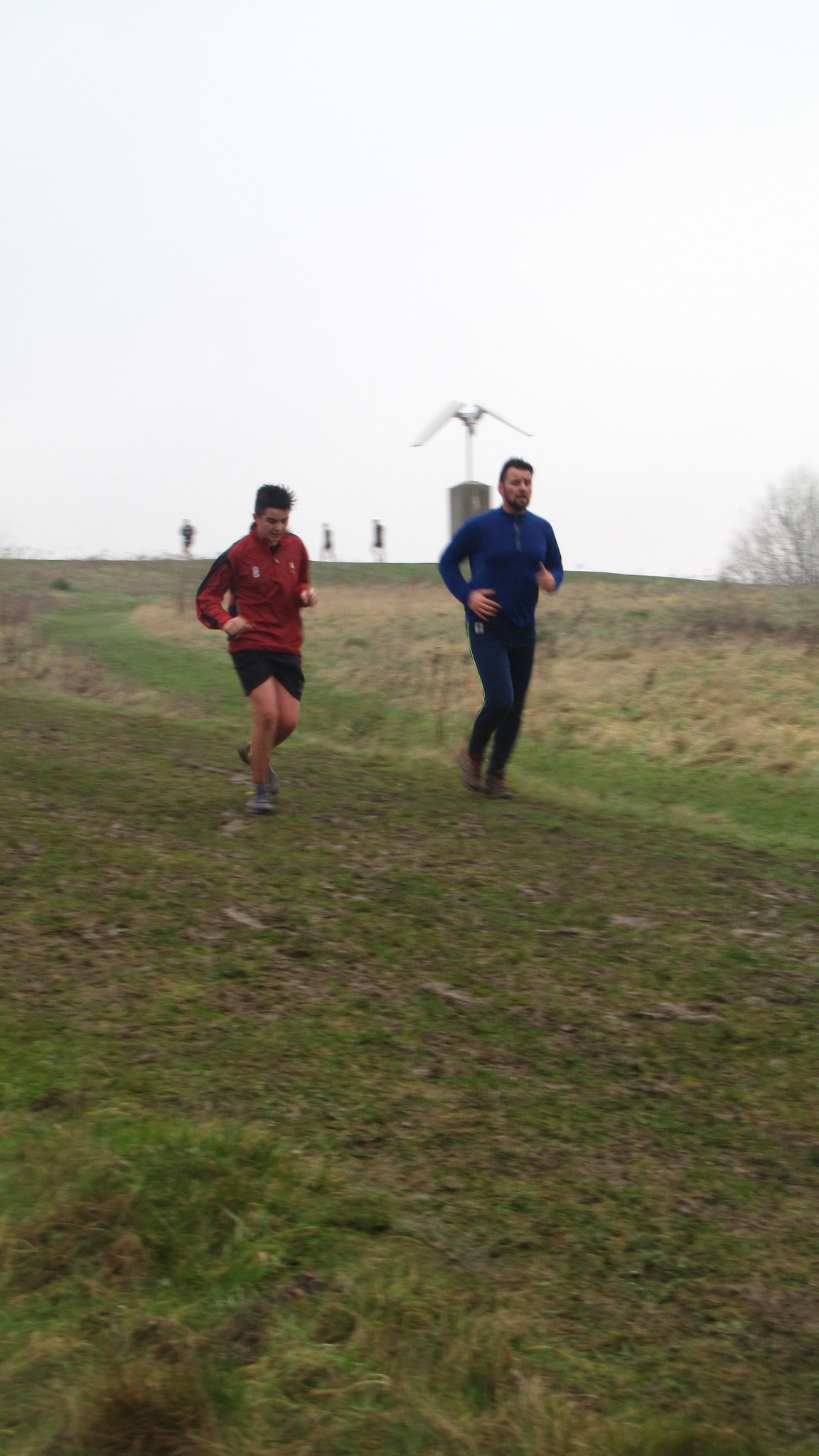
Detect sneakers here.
[238,740,279,792]
[458,748,485,792]
[484,767,515,801]
[251,784,275,814]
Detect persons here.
[370,519,387,563]
[437,457,564,799]
[197,484,317,813]
[320,522,337,561]
[178,518,196,562]
